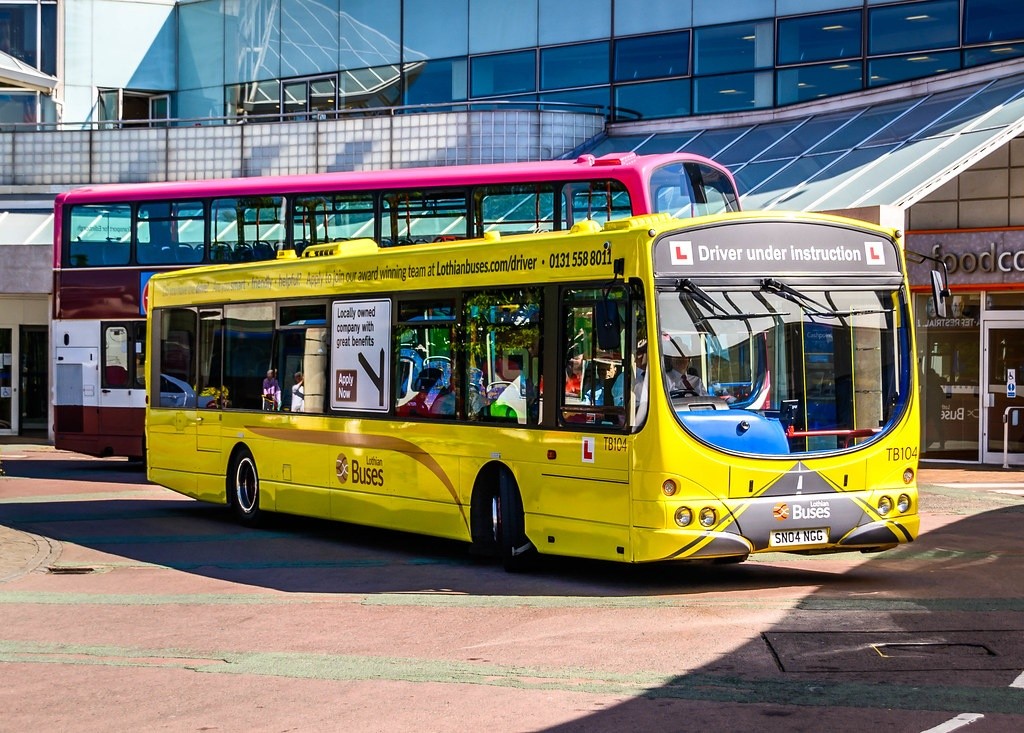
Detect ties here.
[681,374,695,392]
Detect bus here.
[43,149,747,471]
[140,208,953,580]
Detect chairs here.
[121,238,431,265]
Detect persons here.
[291,372,304,412]
[610,343,647,427]
[664,357,708,402]
[565,355,616,407]
[263,370,282,412]
